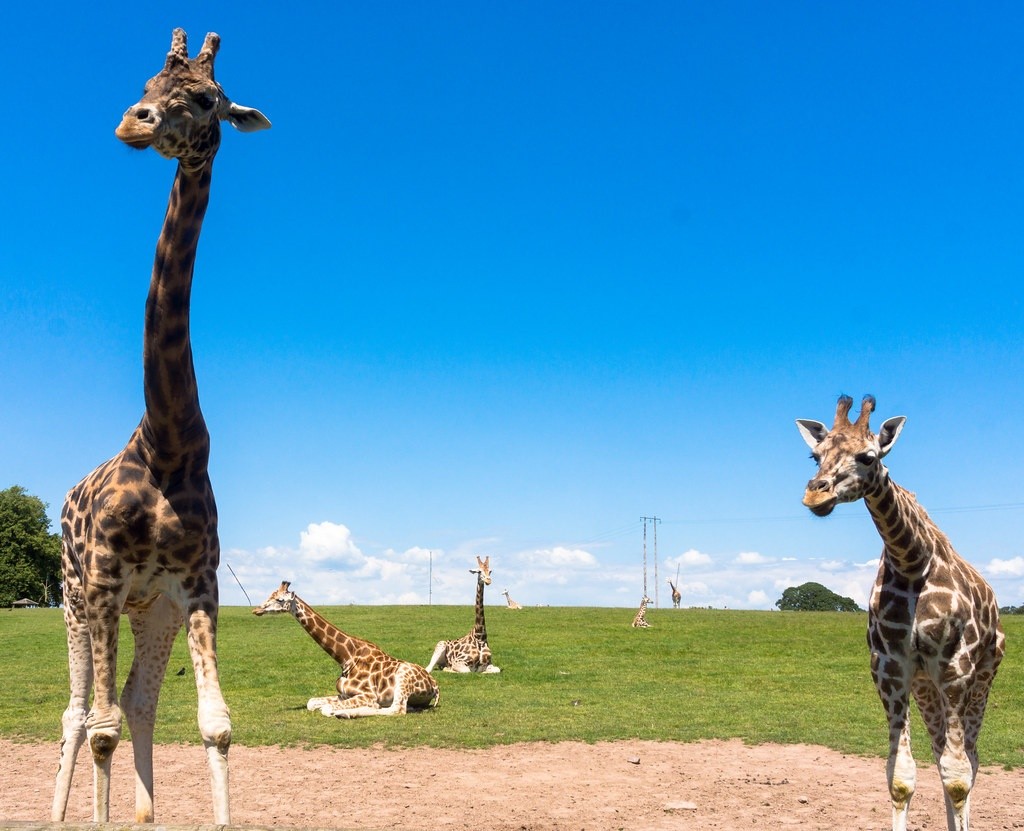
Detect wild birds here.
[176,667,186,676]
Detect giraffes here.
[667,579,681,608]
[793,392,1005,830]
[422,555,502,677]
[251,581,440,721]
[631,594,654,628]
[501,588,523,610]
[49,25,272,825]
